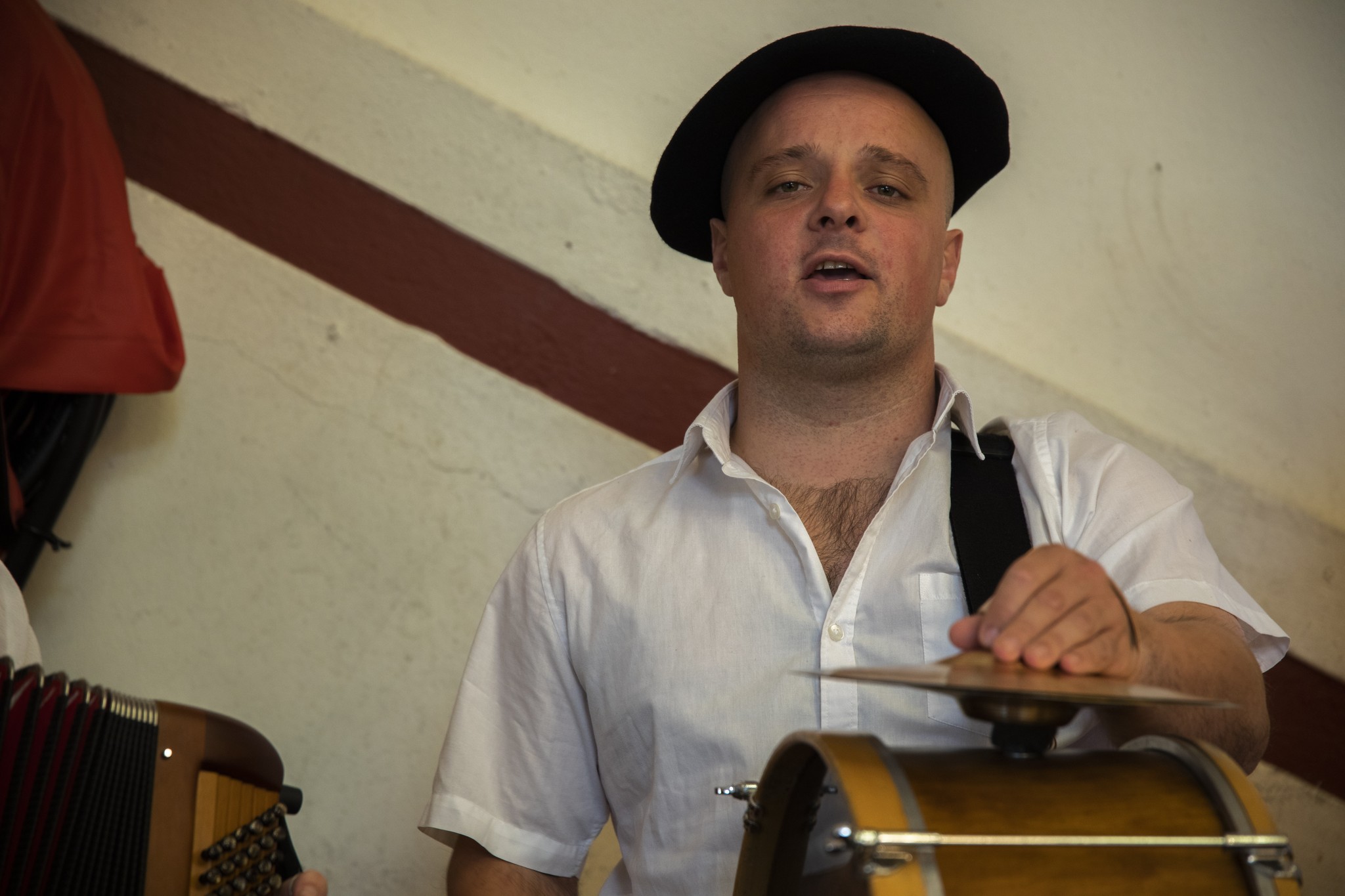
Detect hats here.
[648,24,1011,263]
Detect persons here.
[417,28,1291,895]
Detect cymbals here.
[788,643,1244,734]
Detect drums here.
[713,722,1307,896]
